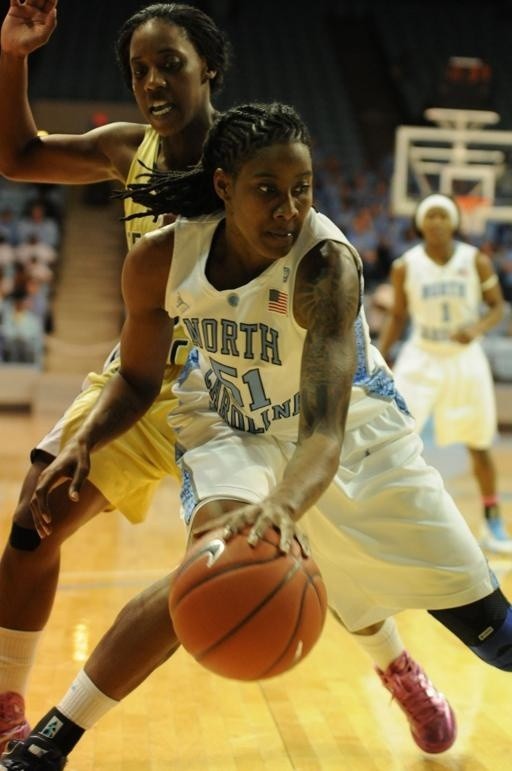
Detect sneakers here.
[0,690,67,771]
[376,650,457,752]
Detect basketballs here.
[169,526,327,680]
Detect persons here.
[0,102,510,771]
[0,2,455,771]
[376,193,510,552]
[314,94,512,378]
[2,176,67,364]
